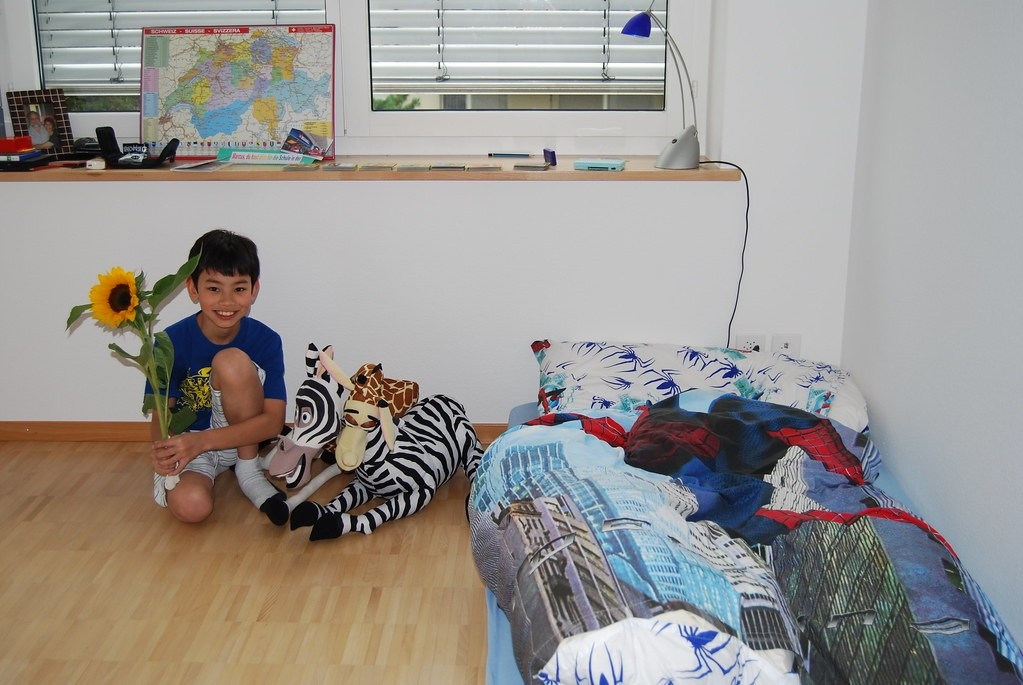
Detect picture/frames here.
[7,87,75,159]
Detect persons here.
[142,229,287,524]
[27,111,63,154]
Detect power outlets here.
[738,335,765,354]
[771,335,803,360]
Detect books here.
[281,128,334,161]
[170,156,233,172]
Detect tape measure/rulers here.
[85,156,106,170]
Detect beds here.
[487,400,1023,685]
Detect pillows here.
[531,338,871,440]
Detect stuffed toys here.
[269,342,485,541]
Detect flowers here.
[65,244,204,438]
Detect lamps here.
[621,0,701,169]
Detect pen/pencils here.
[488,153,536,157]
